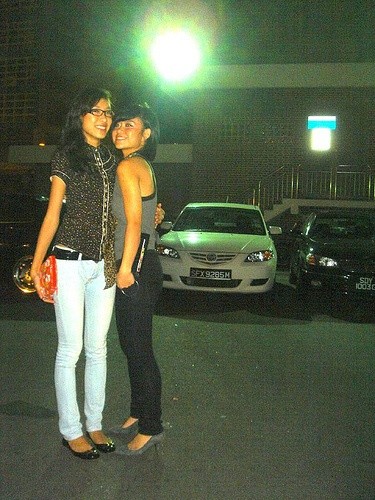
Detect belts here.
[51,248,92,260]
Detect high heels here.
[110,419,139,434]
[115,430,164,456]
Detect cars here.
[290,209,375,307]
[158,203,282,301]
[0,186,69,300]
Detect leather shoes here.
[86,431,115,452]
[62,439,99,459]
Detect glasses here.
[87,109,114,118]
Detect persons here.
[109,102,164,457]
[30,89,165,460]
